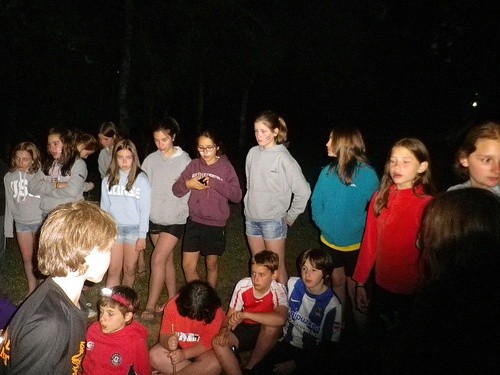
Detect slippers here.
[141,310,156,322]
[156,304,164,313]
[135,269,147,278]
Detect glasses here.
[197,145,216,152]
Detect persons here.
[149,278,225,375]
[0,109,500,335]
[0,199,119,375]
[286,247,343,354]
[211,249,288,375]
[80,286,151,375]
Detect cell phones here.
[198,177,207,186]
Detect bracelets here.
[356,285,364,289]
